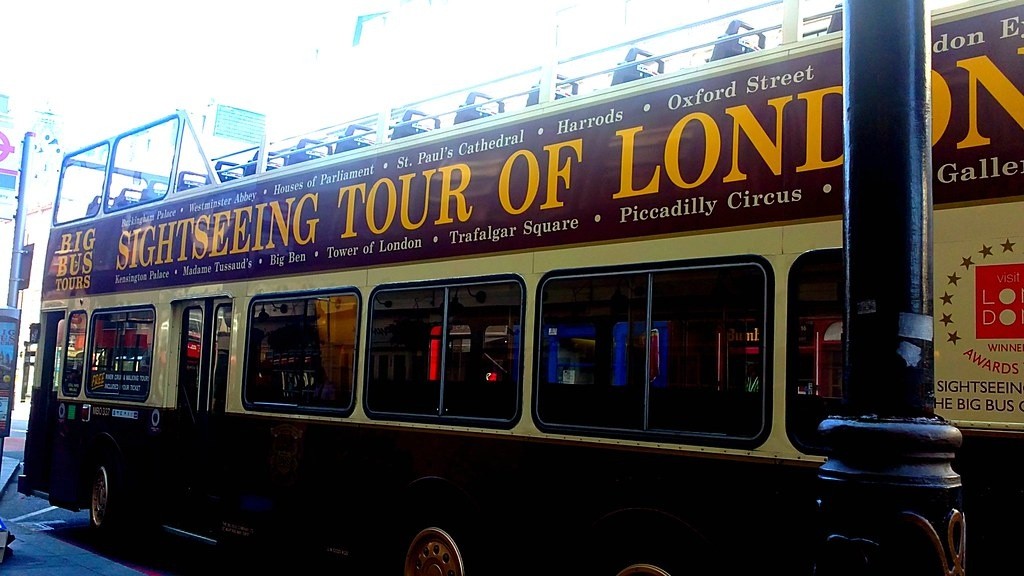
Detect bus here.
[15,0,1024,576]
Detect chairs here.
[213,160,246,185]
[709,18,766,61]
[84,194,115,217]
[335,125,376,154]
[610,47,665,87]
[178,170,209,193]
[287,138,334,166]
[243,150,289,177]
[452,91,506,126]
[391,110,442,141]
[525,73,580,106]
[112,188,142,211]
[142,179,170,201]
